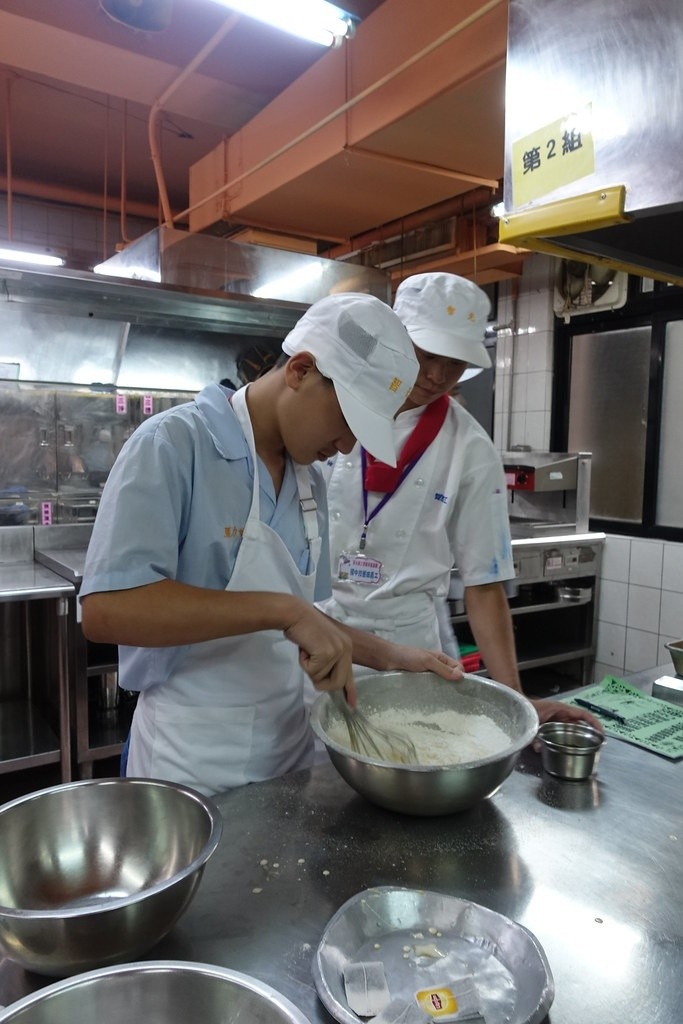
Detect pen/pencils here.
[574,698,626,724]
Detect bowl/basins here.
[310,669,540,816]
[0,776,225,978]
[538,722,606,780]
[0,959,313,1024]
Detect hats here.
[393,272,492,368]
[281,292,420,468]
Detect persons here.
[75,293,464,799]
[312,271,521,755]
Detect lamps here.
[216,0,362,49]
